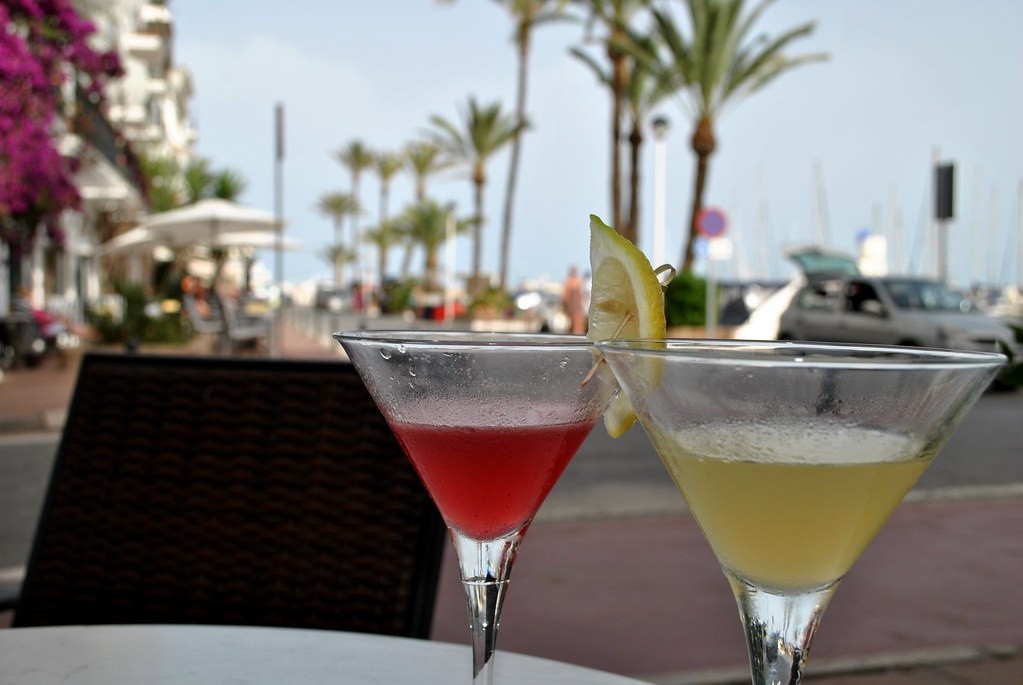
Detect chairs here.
[183,284,269,355]
[0,351,446,640]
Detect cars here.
[773,250,1023,395]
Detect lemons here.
[587,214,667,438]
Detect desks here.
[0,626,645,685]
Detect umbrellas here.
[95,198,300,258]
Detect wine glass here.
[592,338,1008,685]
[333,330,621,685]
